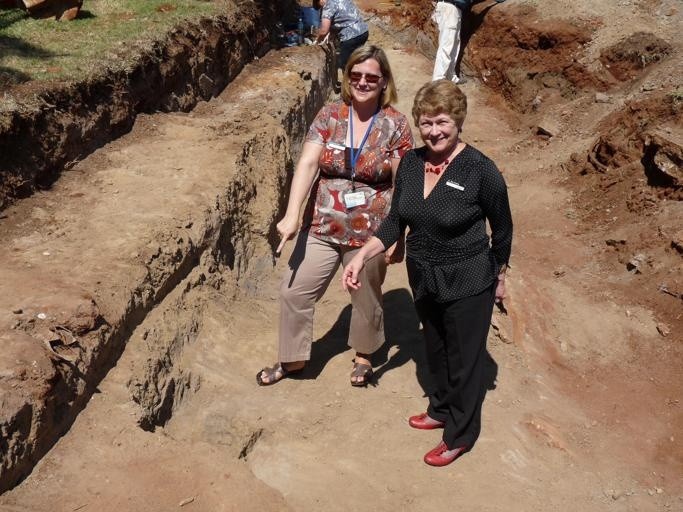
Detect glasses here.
[350,71,385,83]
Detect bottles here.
[296,18,306,45]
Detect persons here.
[428,1,508,87]
[256,45,415,388]
[311,1,368,91]
[341,82,511,468]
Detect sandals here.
[256,362,303,386]
[350,358,375,388]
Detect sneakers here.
[408,411,446,430]
[423,440,470,468]
[456,78,467,83]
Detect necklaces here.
[418,152,457,176]
